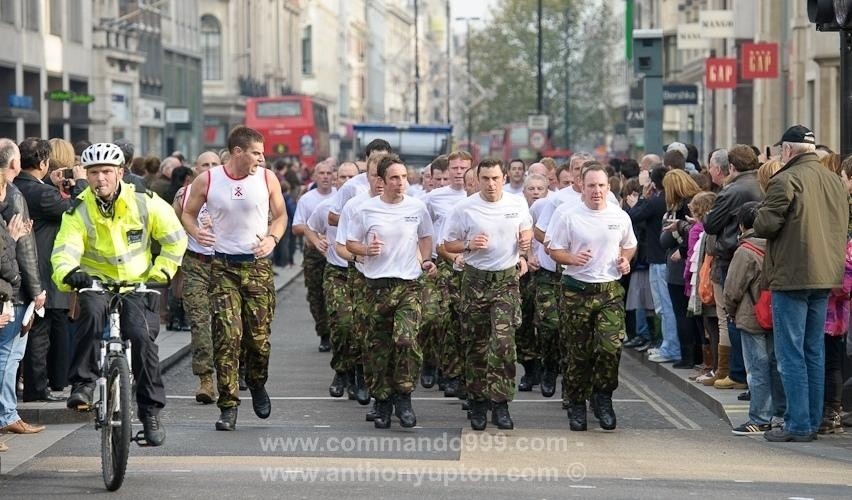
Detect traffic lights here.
[805,0,852,32]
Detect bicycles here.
[60,261,175,494]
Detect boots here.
[438,371,474,418]
[195,372,217,406]
[593,395,618,430]
[568,400,587,433]
[491,401,515,431]
[318,332,332,352]
[517,357,541,391]
[393,392,417,428]
[540,358,560,398]
[375,398,394,430]
[470,398,489,432]
[235,367,247,392]
[366,399,380,423]
[328,367,372,406]
[163,288,195,332]
[248,383,270,418]
[419,359,437,388]
[215,405,238,432]
[704,345,732,386]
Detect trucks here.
[347,121,454,169]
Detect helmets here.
[80,142,126,168]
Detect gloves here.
[64,268,94,292]
[143,278,162,313]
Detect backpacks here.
[740,240,774,330]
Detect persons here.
[0,126,852,454]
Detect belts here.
[561,273,619,292]
[463,262,520,284]
[535,266,564,284]
[184,247,213,265]
[363,275,414,289]
[214,250,259,264]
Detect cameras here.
[63,169,72,178]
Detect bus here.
[243,94,332,175]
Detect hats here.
[649,164,672,190]
[661,141,690,163]
[773,124,817,148]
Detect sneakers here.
[764,427,813,443]
[65,382,94,412]
[730,421,772,435]
[713,375,749,391]
[621,329,715,382]
[136,410,166,446]
[817,409,852,435]
[0,419,46,434]
[736,391,752,401]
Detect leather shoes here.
[33,389,67,403]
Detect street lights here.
[455,16,482,153]
